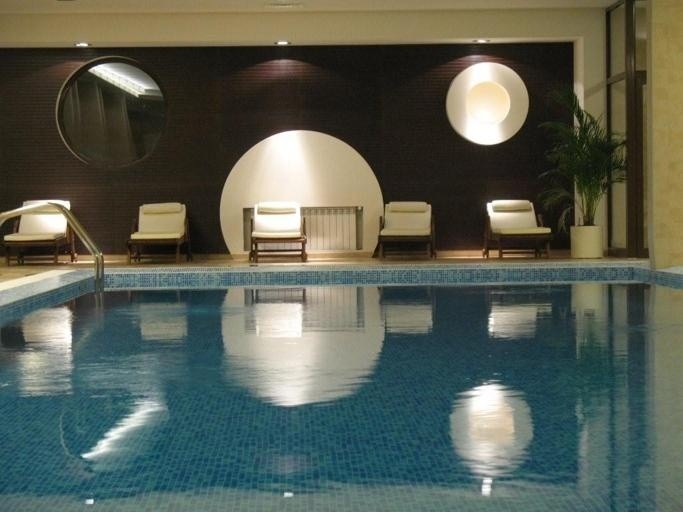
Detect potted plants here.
[532,86,632,260]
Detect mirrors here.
[55,55,167,171]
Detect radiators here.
[257,207,361,253]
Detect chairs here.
[124,202,195,264]
[377,199,553,259]
[2,200,78,268]
[249,202,306,269]
